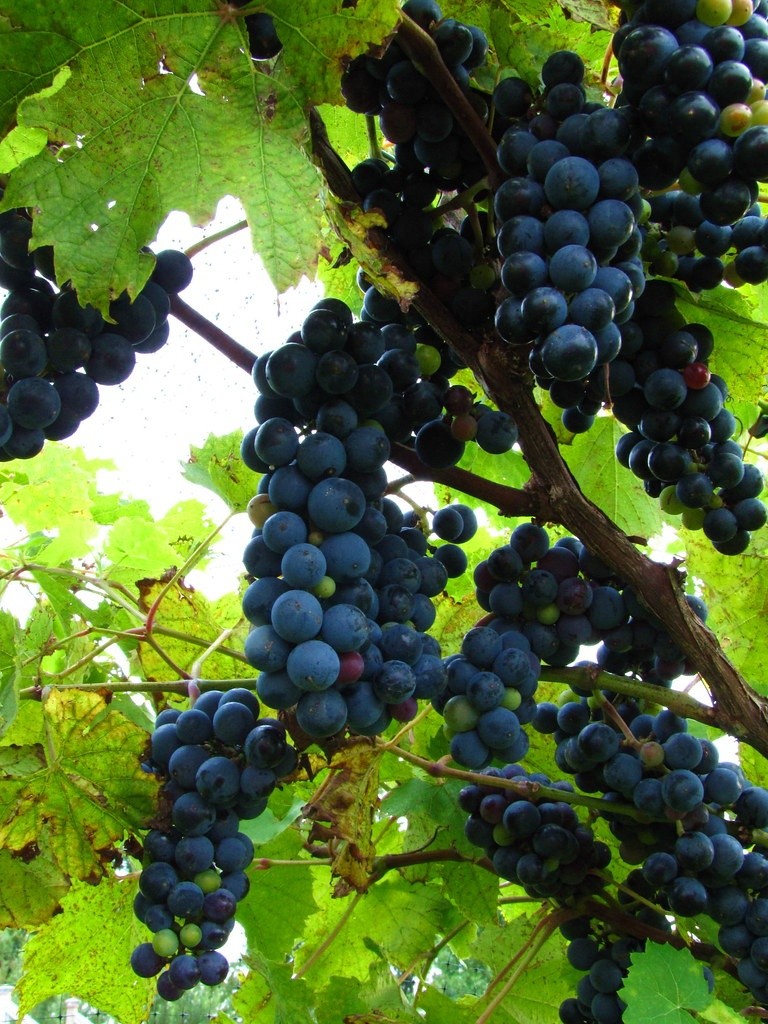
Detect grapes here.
[0,1,768,1022]
